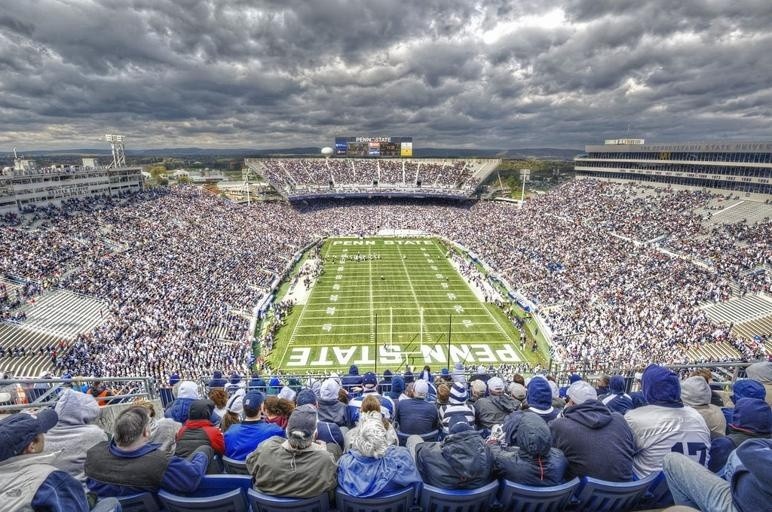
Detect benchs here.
[538,177,772,367]
[0,199,273,380]
[403,157,502,191]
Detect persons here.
[0,157,771,511]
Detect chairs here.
[0,374,771,512]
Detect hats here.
[226,389,245,414]
[362,371,377,389]
[548,381,559,398]
[566,381,597,402]
[471,377,526,400]
[412,378,429,398]
[286,403,316,437]
[1,410,58,461]
[448,415,474,434]
[297,389,314,404]
